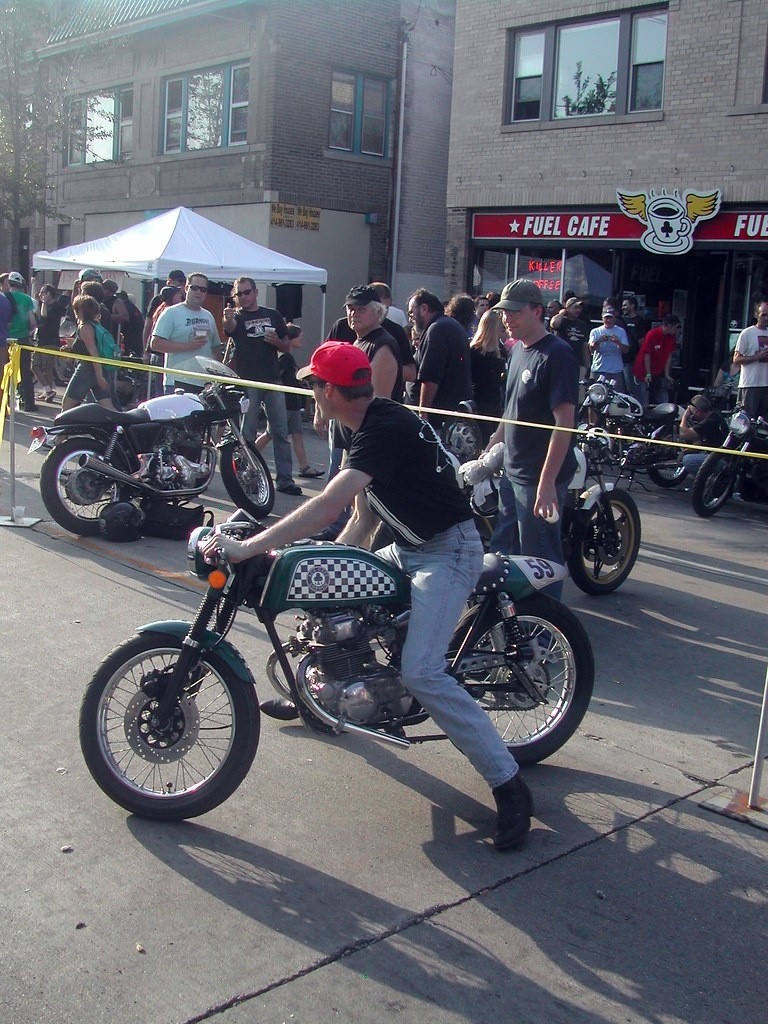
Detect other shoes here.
[34,390,56,402]
[276,483,303,495]
[19,405,39,412]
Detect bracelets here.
[646,373,652,377]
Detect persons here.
[308,281,681,540]
[478,277,579,663]
[0,269,326,495]
[202,340,534,850]
[679,299,768,480]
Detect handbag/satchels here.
[140,497,215,541]
[146,335,163,355]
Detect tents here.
[33,206,328,431]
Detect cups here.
[264,327,276,340]
[538,502,559,524]
[196,330,207,341]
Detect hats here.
[340,285,381,310]
[8,272,22,283]
[295,341,372,385]
[566,297,584,309]
[101,279,119,295]
[602,306,616,318]
[79,269,100,282]
[161,286,182,302]
[490,278,542,310]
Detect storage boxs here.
[141,499,204,540]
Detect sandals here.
[298,466,324,477]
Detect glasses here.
[234,289,254,297]
[686,400,700,410]
[188,285,207,293]
[304,380,319,390]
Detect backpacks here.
[77,320,121,371]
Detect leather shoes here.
[492,771,535,851]
[260,698,300,720]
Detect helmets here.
[98,502,145,543]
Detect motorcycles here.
[78,508,597,819]
[693,400,768,517]
[26,356,275,537]
[439,400,641,596]
[578,375,696,492]
